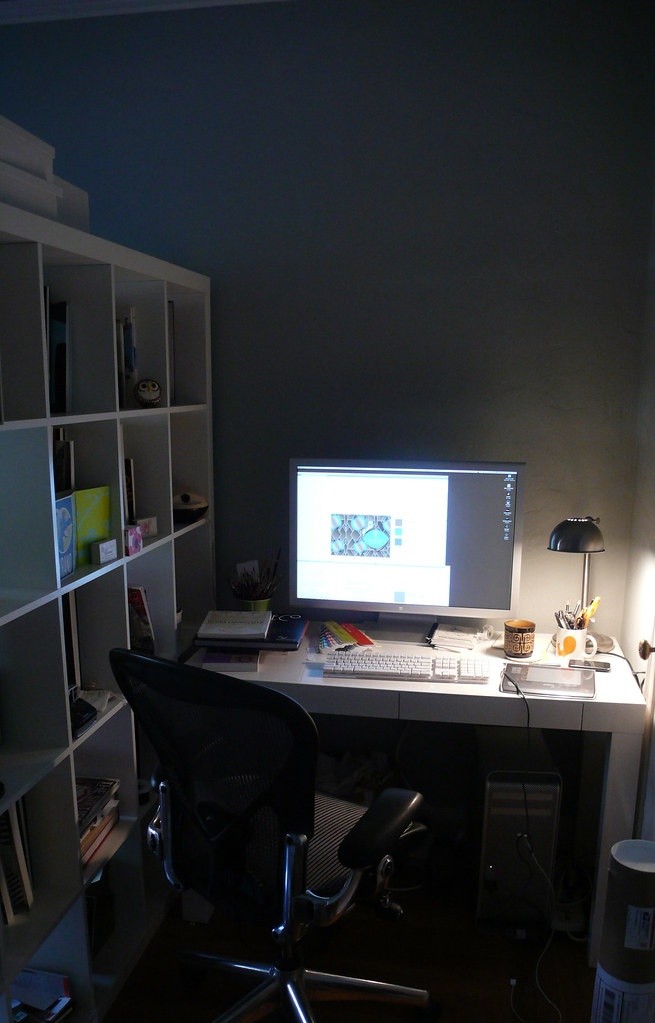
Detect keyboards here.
[322,650,489,684]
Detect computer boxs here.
[472,748,564,947]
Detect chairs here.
[109,647,430,1023]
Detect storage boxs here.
[125,525,144,557]
[55,492,76,581]
[75,481,109,568]
[0,162,63,223]
[0,115,55,185]
[132,516,157,539]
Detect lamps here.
[547,516,614,650]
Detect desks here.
[184,617,647,975]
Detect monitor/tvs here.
[287,457,527,647]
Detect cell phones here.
[569,659,611,672]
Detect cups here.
[504,619,536,658]
[555,626,597,664]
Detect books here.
[116,304,137,411]
[52,428,110,579]
[128,584,155,657]
[202,650,260,672]
[8,968,72,1023]
[194,614,309,651]
[0,799,34,924]
[44,286,70,418]
[75,775,121,866]
[120,424,135,525]
[197,611,272,639]
[427,623,474,653]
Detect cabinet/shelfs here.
[0,199,213,1023]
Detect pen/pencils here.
[554,596,601,630]
[223,548,282,600]
